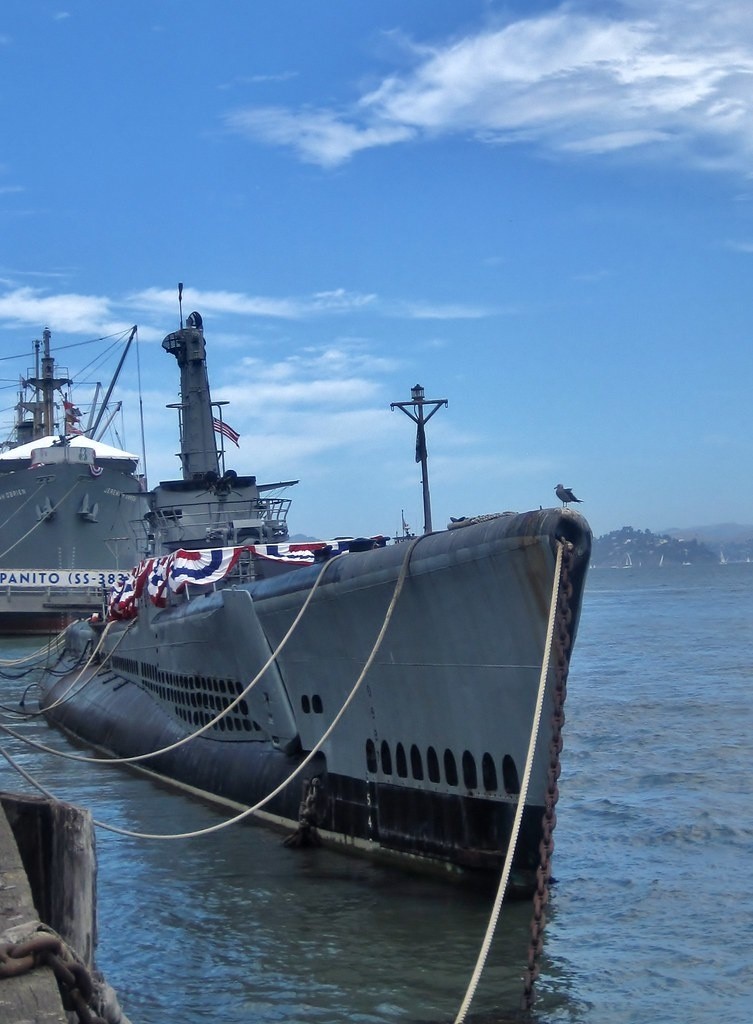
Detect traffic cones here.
[622,553,632,569]
[719,552,727,564]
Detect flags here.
[63,401,82,434]
[213,417,240,448]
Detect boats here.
[39,282,592,899]
[0,326,151,636]
